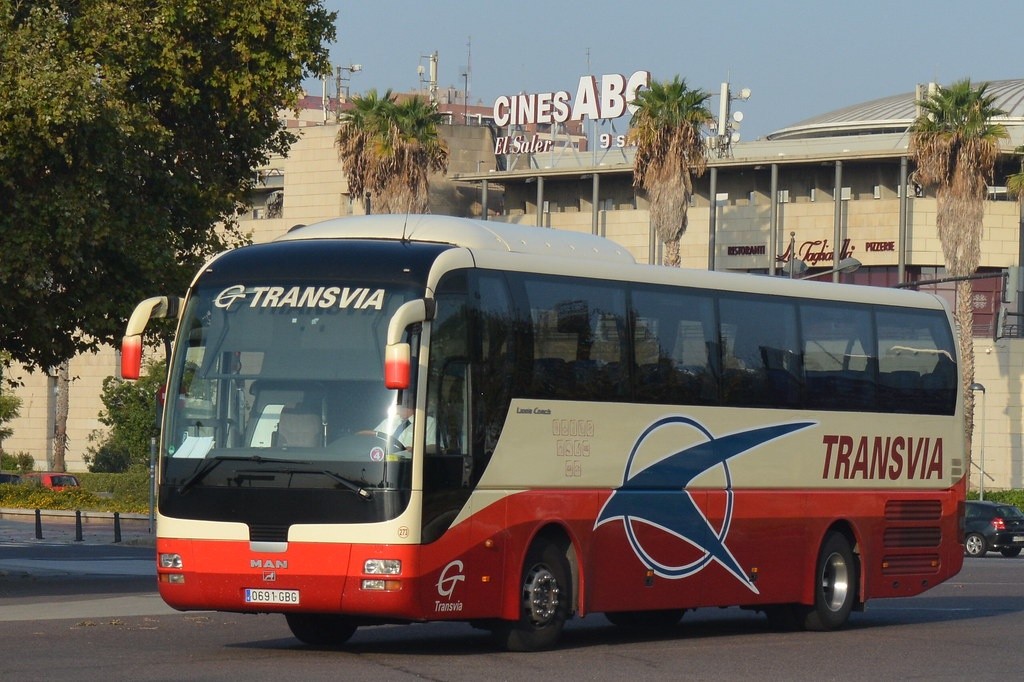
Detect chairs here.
[490,347,922,405]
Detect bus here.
[121,214,967,653]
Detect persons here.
[373,390,449,456]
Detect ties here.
[391,420,411,441]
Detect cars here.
[18,471,82,494]
[0,469,25,485]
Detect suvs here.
[964,500,1024,558]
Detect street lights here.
[335,65,363,125]
[969,382,986,500]
[417,49,439,115]
